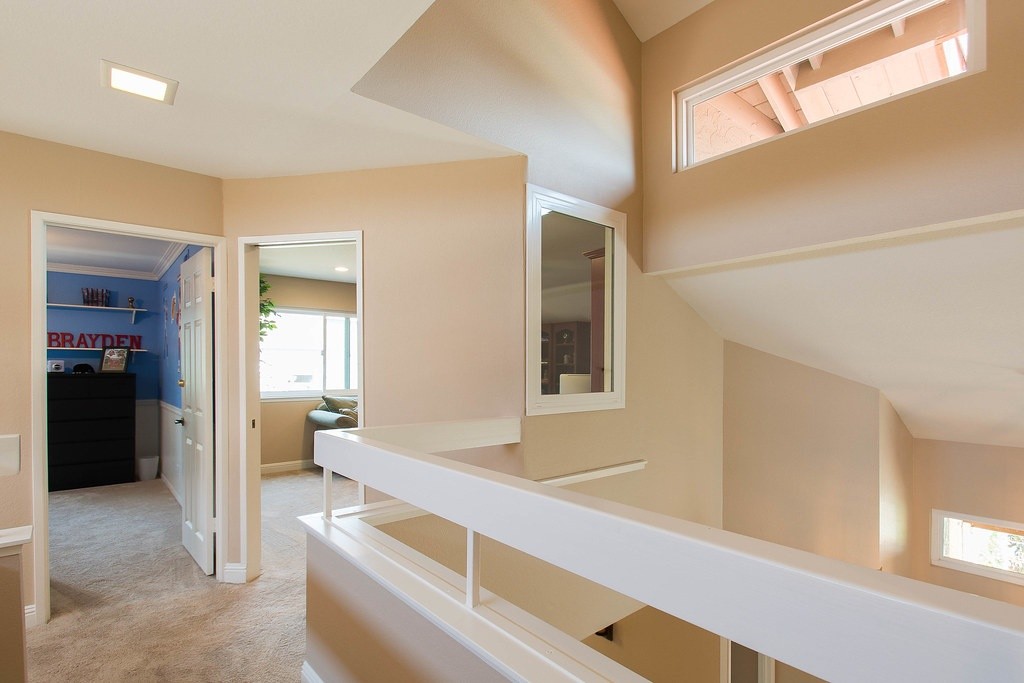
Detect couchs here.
[306,396,357,479]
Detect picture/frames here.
[97,346,130,372]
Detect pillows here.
[322,396,357,413]
[339,406,357,420]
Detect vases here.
[138,455,160,481]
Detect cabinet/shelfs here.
[46,288,148,352]
[553,321,590,393]
[47,372,138,492]
[541,323,554,395]
[583,248,605,392]
